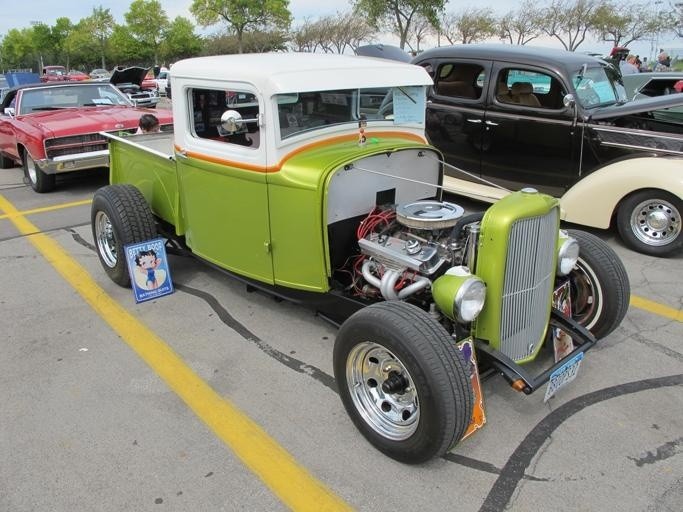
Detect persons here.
[136,250,163,290]
[627,49,669,72]
[136,114,162,135]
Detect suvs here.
[352,42,681,262]
[89,53,633,467]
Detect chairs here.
[76,92,96,106]
[496,81,542,108]
[436,81,474,99]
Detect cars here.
[150,67,169,97]
[1,80,174,194]
[115,81,161,108]
[0,73,41,106]
[40,65,111,84]
